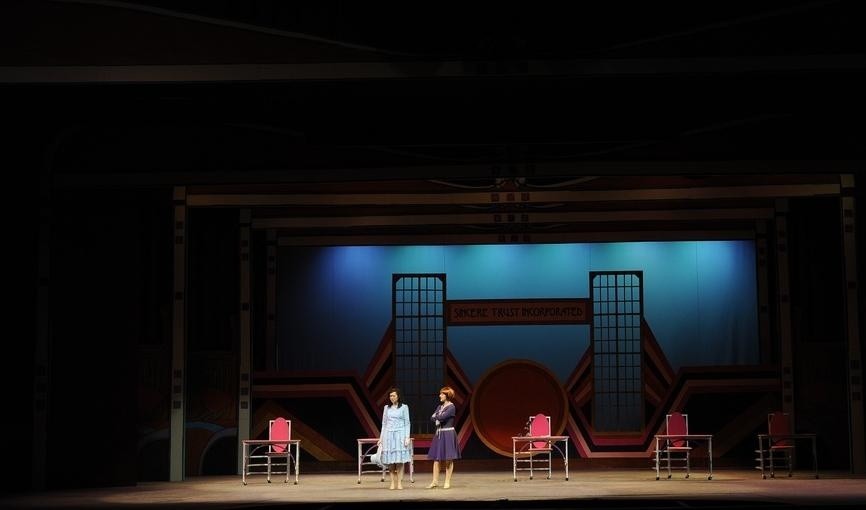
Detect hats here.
[370,444,382,465]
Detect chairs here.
[766,412,797,478]
[528,414,553,479]
[267,417,291,483]
[664,411,690,478]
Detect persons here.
[375,385,411,490]
[423,386,463,490]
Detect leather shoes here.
[426,481,440,489]
[390,480,395,489]
[444,480,450,488]
[398,480,403,489]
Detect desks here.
[238,439,303,486]
[652,434,714,481]
[356,437,415,484]
[754,433,818,479]
[511,435,571,482]
